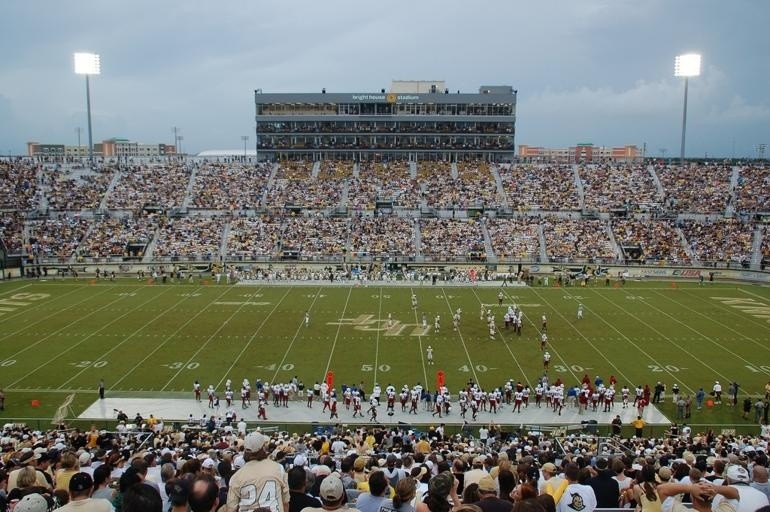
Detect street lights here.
[72,51,102,166]
[673,52,702,164]
[171,125,181,146]
[74,125,87,158]
[176,135,184,152]
[241,135,249,166]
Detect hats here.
[473,454,488,465]
[294,454,343,501]
[69,472,93,491]
[202,458,215,468]
[539,463,557,473]
[13,493,54,512]
[727,453,750,482]
[79,450,112,464]
[479,477,497,491]
[658,466,671,479]
[354,456,365,470]
[683,451,695,464]
[412,461,453,497]
[234,432,270,468]
[20,434,67,464]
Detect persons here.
[0,151,770,286]
[409,290,552,373]
[192,374,770,424]
[0,411,769,511]
[305,311,310,327]
[257,108,514,163]
[99,378,105,399]
[577,304,584,320]
